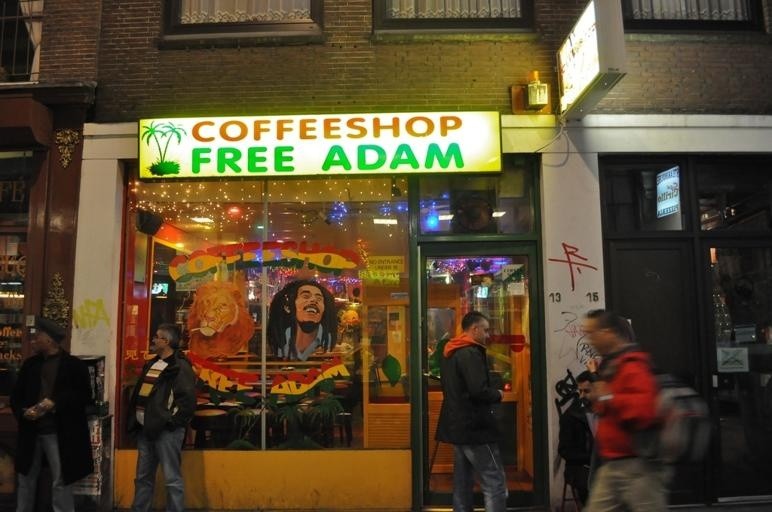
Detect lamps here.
[523,67,549,112]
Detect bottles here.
[585,358,608,418]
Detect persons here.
[435,310,508,512]
[557,365,605,505]
[268,277,338,359]
[580,309,672,512]
[127,323,197,511]
[9,317,96,512]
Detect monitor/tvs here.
[151,283,169,297]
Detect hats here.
[29,318,66,344]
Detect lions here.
[185,280,257,359]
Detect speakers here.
[135,207,163,235]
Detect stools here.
[187,380,354,450]
